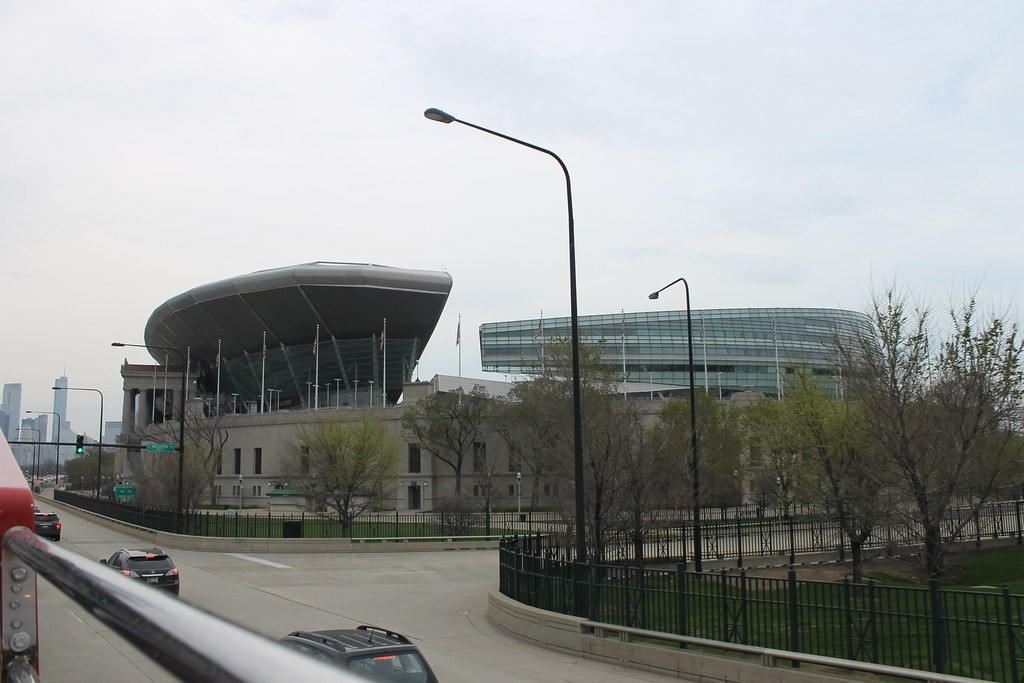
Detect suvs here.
[274,624,440,683]
[33,506,62,542]
[98,547,180,599]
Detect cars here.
[24,473,67,494]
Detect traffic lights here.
[76,435,85,454]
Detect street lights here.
[647,277,705,581]
[16,428,41,481]
[112,342,188,515]
[422,106,589,563]
[14,437,36,490]
[25,411,61,485]
[52,386,104,500]
